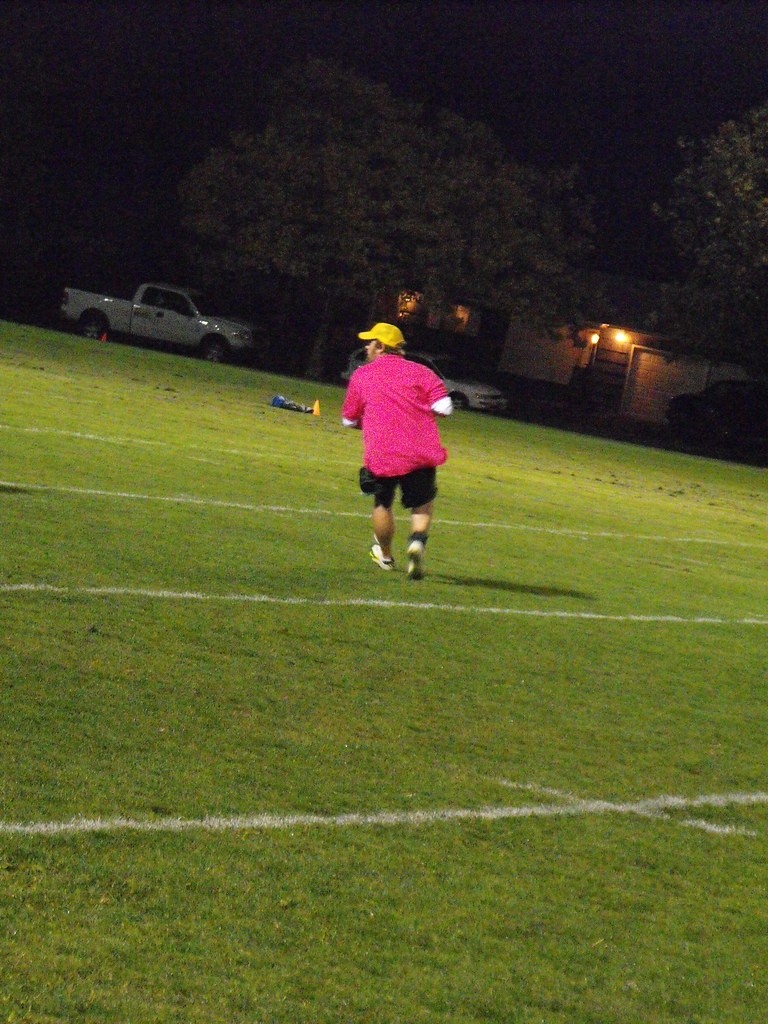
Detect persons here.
[339,322,453,580]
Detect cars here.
[664,379,768,463]
[341,346,509,413]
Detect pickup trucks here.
[60,282,254,362]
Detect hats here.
[357,323,405,349]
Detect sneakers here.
[406,549,425,581]
[369,543,395,570]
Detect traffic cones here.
[312,399,320,416]
[101,333,107,342]
